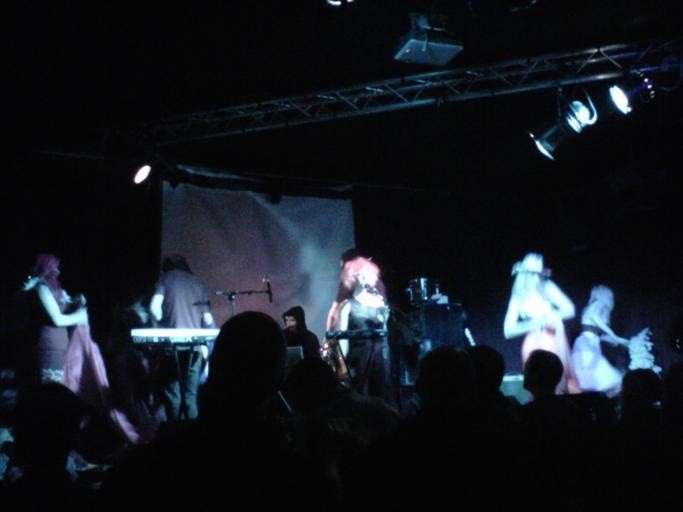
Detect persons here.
[0,250,682,512]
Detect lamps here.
[529,35,673,160]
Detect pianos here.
[128,325,220,345]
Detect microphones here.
[262,276,273,303]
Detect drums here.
[380,340,435,389]
[407,276,436,309]
